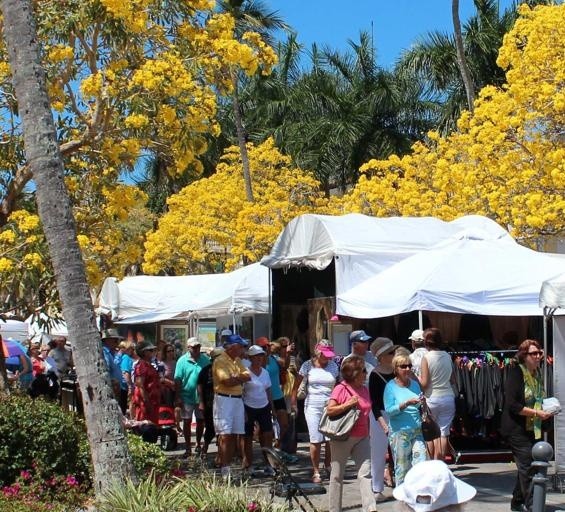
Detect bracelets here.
[535,409,537,417]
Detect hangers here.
[448,350,515,366]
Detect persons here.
[210,329,344,483]
[327,329,477,511]
[503,339,551,512]
[103,329,216,463]
[2,329,75,404]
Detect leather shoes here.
[511,504,531,512]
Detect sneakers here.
[312,474,322,483]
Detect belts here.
[216,392,242,398]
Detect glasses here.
[528,352,543,355]
[395,364,412,369]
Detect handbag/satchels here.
[422,422,441,441]
[318,406,361,441]
[297,377,308,400]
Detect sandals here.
[184,449,191,458]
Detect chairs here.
[260,447,327,512]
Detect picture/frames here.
[193,321,217,347]
[155,320,190,361]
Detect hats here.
[317,345,336,358]
[187,329,270,358]
[392,460,477,512]
[350,330,372,342]
[101,329,124,342]
[408,330,424,342]
[375,341,399,359]
[138,343,157,351]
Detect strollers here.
[152,383,178,452]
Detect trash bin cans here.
[60,380,83,414]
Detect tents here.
[0,309,108,355]
